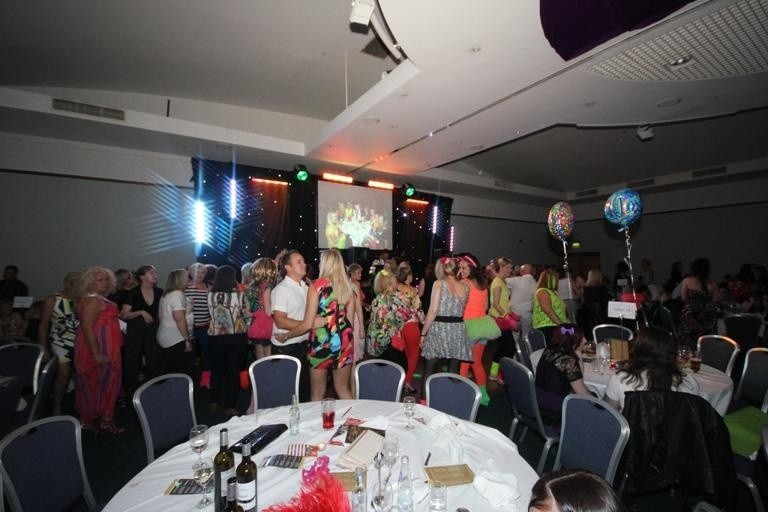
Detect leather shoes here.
[208,402,222,416]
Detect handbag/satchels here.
[391,335,404,352]
[246,282,272,341]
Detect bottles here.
[349,468,368,512]
[287,395,300,434]
[214,429,236,512]
[236,439,258,512]
[223,477,243,512]
[397,456,413,512]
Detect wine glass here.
[402,396,415,430]
[191,457,214,508]
[189,425,213,470]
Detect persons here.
[1,248,768,511]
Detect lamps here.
[293,163,310,182]
[401,181,416,197]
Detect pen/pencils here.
[424,452,432,466]
[341,406,353,419]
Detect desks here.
[108,395,539,510]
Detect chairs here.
[354,358,400,403]
[249,354,302,409]
[0,415,96,511]
[131,372,198,461]
[425,372,482,420]
[1,302,128,444]
[553,392,631,487]
[498,300,766,472]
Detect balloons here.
[548,201,575,243]
[603,187,644,230]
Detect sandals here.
[80,423,100,434]
[99,420,126,435]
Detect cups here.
[381,436,399,466]
[429,481,447,512]
[320,398,335,430]
[373,481,394,512]
